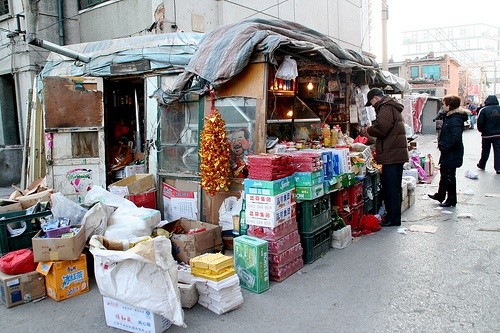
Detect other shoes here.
[428,193,445,204]
[477,163,485,170]
[439,200,457,207]
[380,221,401,226]
[496,169,500,174]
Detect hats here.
[364,88,382,107]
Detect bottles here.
[323,124,331,148]
[331,126,338,148]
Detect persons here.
[476,103,483,116]
[433,105,444,140]
[428,96,471,208]
[364,88,409,227]
[477,95,500,174]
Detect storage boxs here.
[0,144,385,333]
